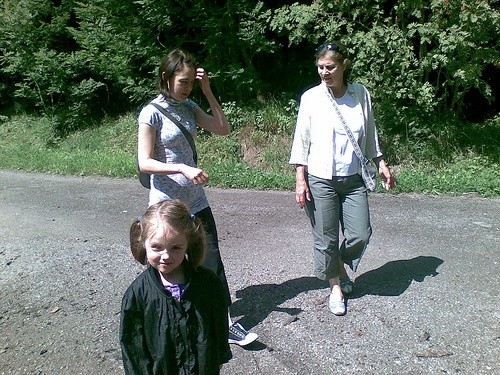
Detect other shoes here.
[340,274,352,294]
[227,322,258,346]
[328,289,345,316]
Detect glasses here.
[317,43,345,58]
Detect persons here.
[289,43,394,316]
[118,198,233,375]
[138,49,259,346]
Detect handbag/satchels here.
[362,158,377,192]
[137,165,151,189]
